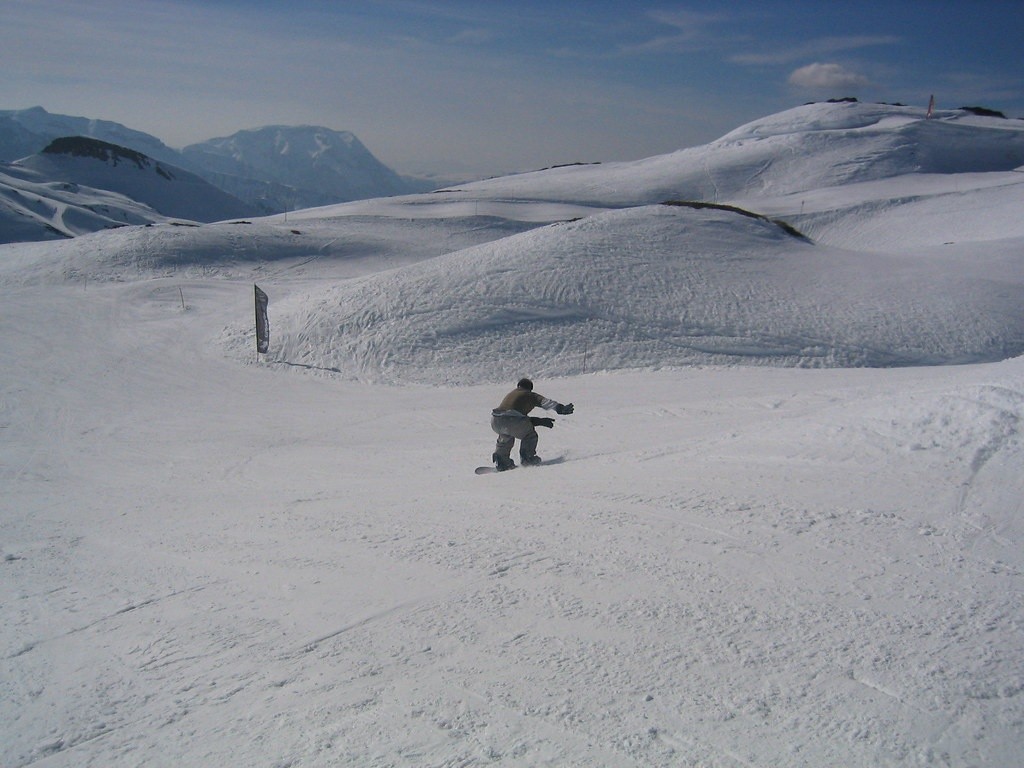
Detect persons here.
[490,378,575,471]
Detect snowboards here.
[475,454,568,475]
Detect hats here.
[517,378,533,390]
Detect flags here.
[255,285,269,354]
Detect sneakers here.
[521,455,543,466]
[496,458,516,471]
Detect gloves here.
[531,415,556,430]
[557,402,575,415]
